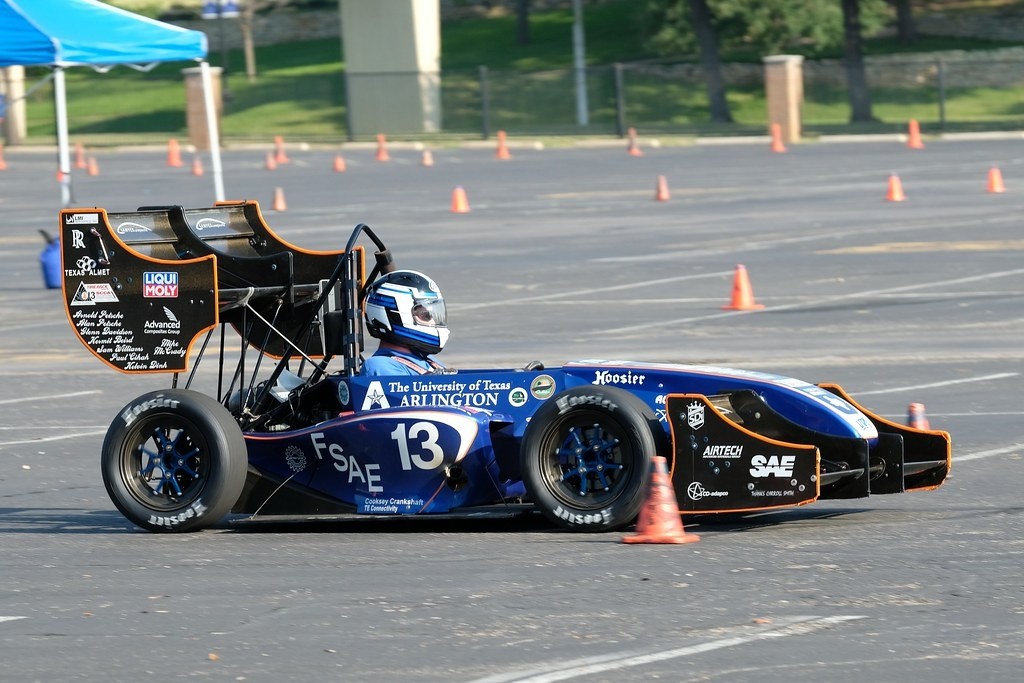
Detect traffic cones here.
[333,155,346,173]
[719,264,765,310]
[496,130,511,160]
[449,187,471,214]
[422,151,433,167]
[985,168,1006,194]
[885,174,904,202]
[191,158,204,177]
[274,136,289,163]
[770,124,786,153]
[377,134,389,161]
[265,152,277,171]
[627,127,643,156]
[906,119,924,150]
[274,188,287,212]
[621,456,700,545]
[0,143,100,177]
[655,175,669,201]
[167,139,184,168]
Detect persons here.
[358,270,451,377]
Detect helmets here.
[364,270,450,354]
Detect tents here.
[0,0,226,205]
